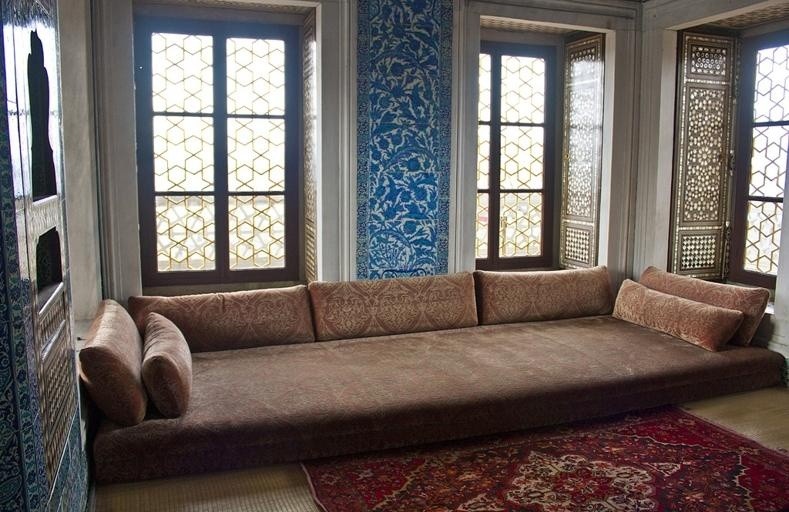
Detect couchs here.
[74,263,789,484]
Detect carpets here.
[300,403,789,512]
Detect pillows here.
[79,299,146,428]
[120,283,315,348]
[614,279,744,351]
[473,264,616,326]
[310,271,478,340]
[638,265,770,346]
[141,311,192,418]
